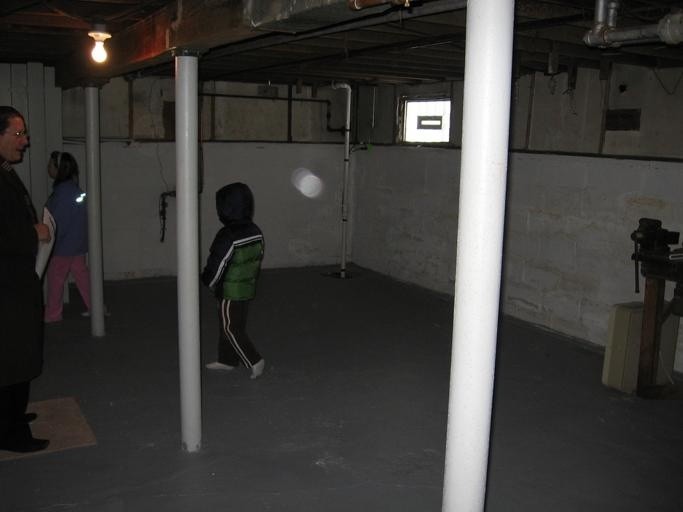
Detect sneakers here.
[206,362,234,371]
[250,359,266,380]
[82,306,112,318]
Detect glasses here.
[5,132,26,136]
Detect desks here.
[630,252,683,399]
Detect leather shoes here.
[0,413,49,452]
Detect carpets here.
[0,396,98,462]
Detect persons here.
[201,182,266,379]
[0,106,110,451]
